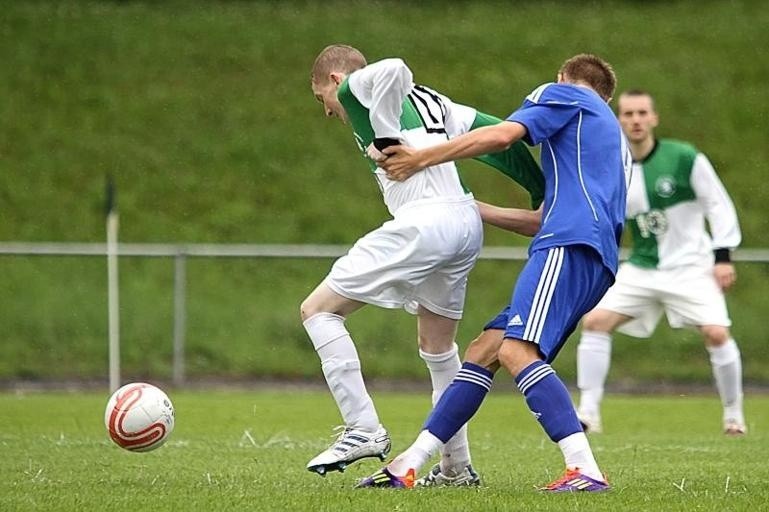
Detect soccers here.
[104,382,175,453]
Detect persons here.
[572,88,751,437]
[298,41,546,488]
[353,51,632,493]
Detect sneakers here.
[724,420,744,436]
[306,424,392,476]
[415,463,480,486]
[354,467,415,490]
[539,469,610,492]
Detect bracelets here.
[714,248,730,263]
[372,136,402,156]
